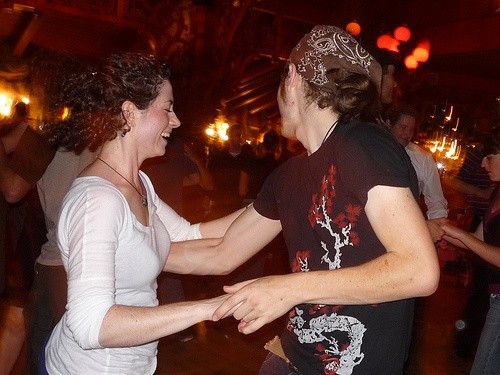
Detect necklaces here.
[320,118,338,145]
[97,157,148,206]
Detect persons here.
[433,133,500,375]
[140,126,299,342]
[387,105,449,243]
[0,102,104,375]
[163,24,440,375]
[39,54,259,375]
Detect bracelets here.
[246,205,248,209]
